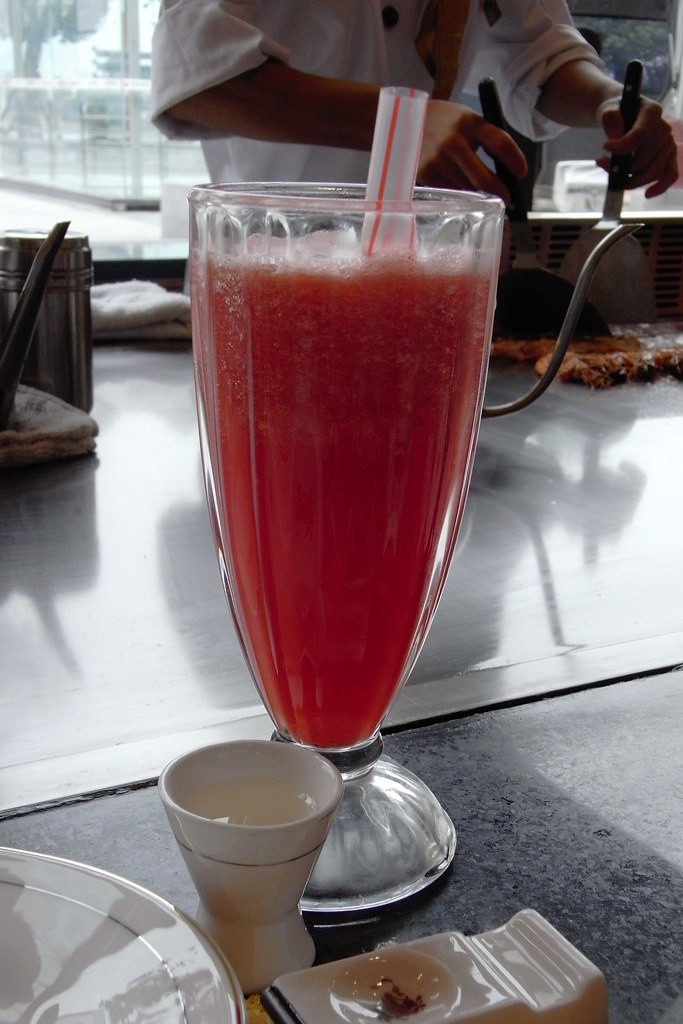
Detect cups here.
[160,742,342,992]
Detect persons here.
[149,0,679,240]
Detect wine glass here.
[189,183,505,913]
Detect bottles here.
[0,229,93,416]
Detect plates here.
[0,846,245,1023]
